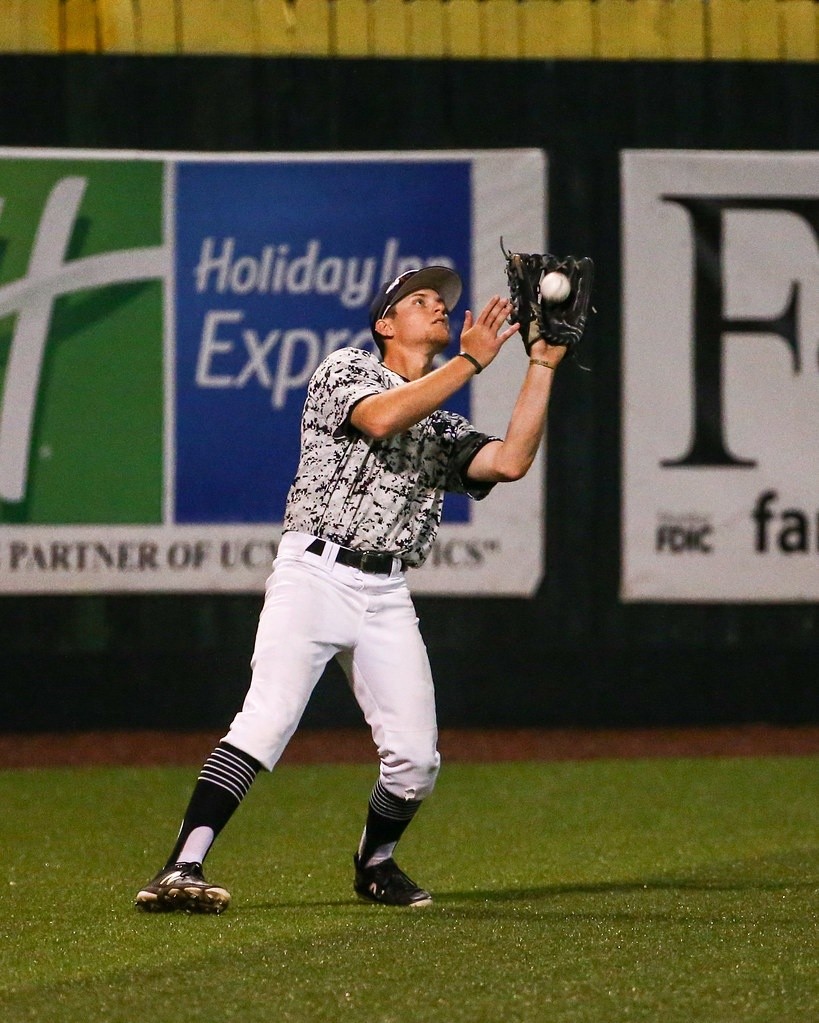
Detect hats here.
[367,264,462,343]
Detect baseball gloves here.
[499,235,596,357]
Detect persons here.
[136,265,567,913]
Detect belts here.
[305,539,408,575]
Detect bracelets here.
[457,352,482,374]
[530,359,558,369]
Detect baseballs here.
[539,271,571,303]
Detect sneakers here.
[136,860,230,914]
[350,852,434,908]
